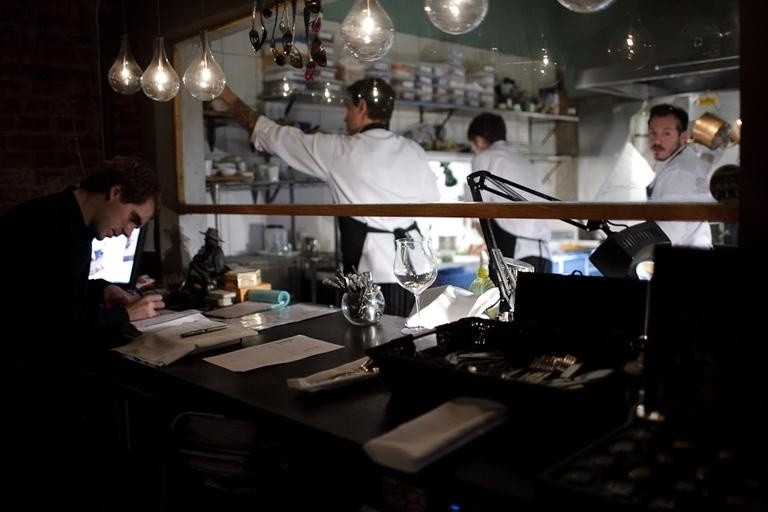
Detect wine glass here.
[392,239,438,337]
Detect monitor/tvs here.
[88,227,147,289]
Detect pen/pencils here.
[130,282,144,298]
[181,325,228,338]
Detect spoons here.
[248,1,292,53]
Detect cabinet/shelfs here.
[263,85,576,169]
[203,108,253,183]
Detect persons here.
[219,77,440,318]
[183,226,227,282]
[0,156,167,512]
[629,104,713,250]
[461,112,553,317]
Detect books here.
[123,306,243,355]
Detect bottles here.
[304,236,318,252]
[262,223,294,254]
[339,290,385,327]
[496,68,569,116]
[409,122,434,151]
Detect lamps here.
[340,0,397,64]
[107,0,143,95]
[558,0,610,13]
[140,0,181,101]
[422,0,487,35]
[182,1,227,101]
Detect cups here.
[257,157,283,181]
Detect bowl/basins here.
[204,155,253,177]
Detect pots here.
[685,112,730,149]
